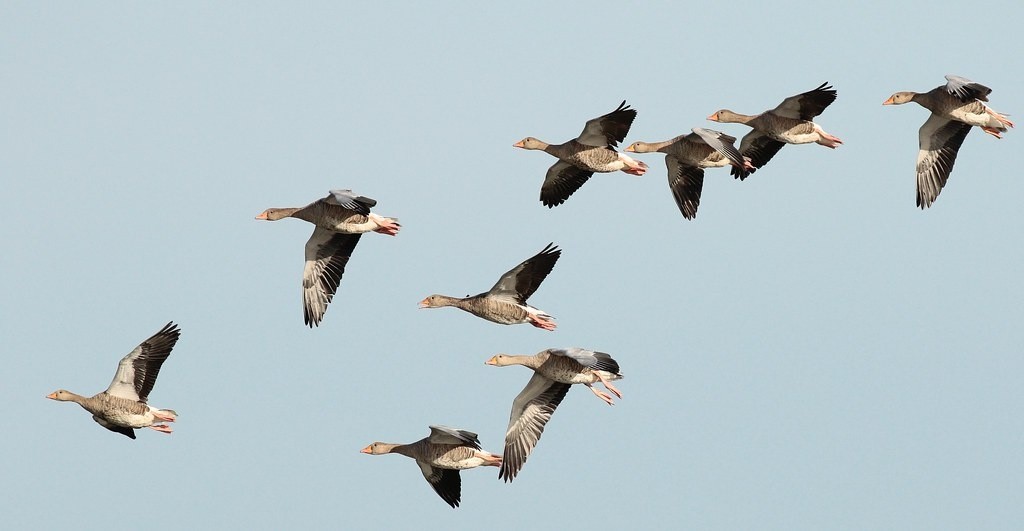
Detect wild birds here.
[46,75,1015,509]
